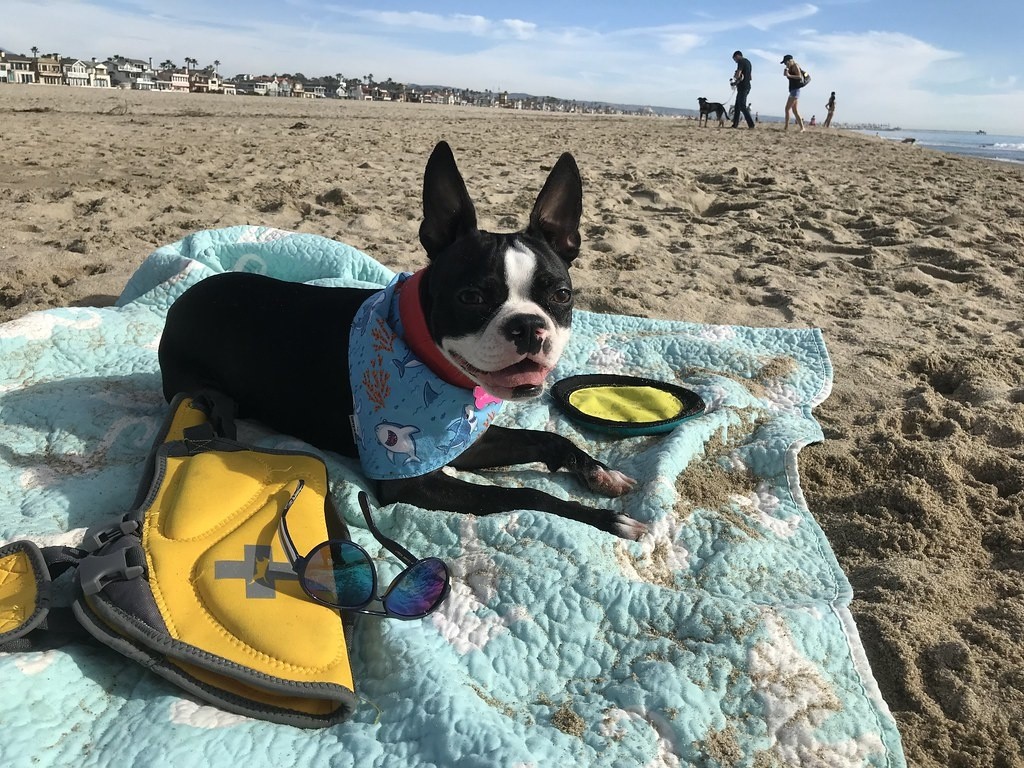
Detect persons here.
[823,92,835,127]
[731,51,754,128]
[811,115,815,125]
[756,112,758,122]
[781,55,805,132]
[746,103,751,113]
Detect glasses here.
[278,480,451,621]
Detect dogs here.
[157,140,653,543]
[696,97,730,128]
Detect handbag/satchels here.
[795,68,810,89]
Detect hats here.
[781,54,793,64]
